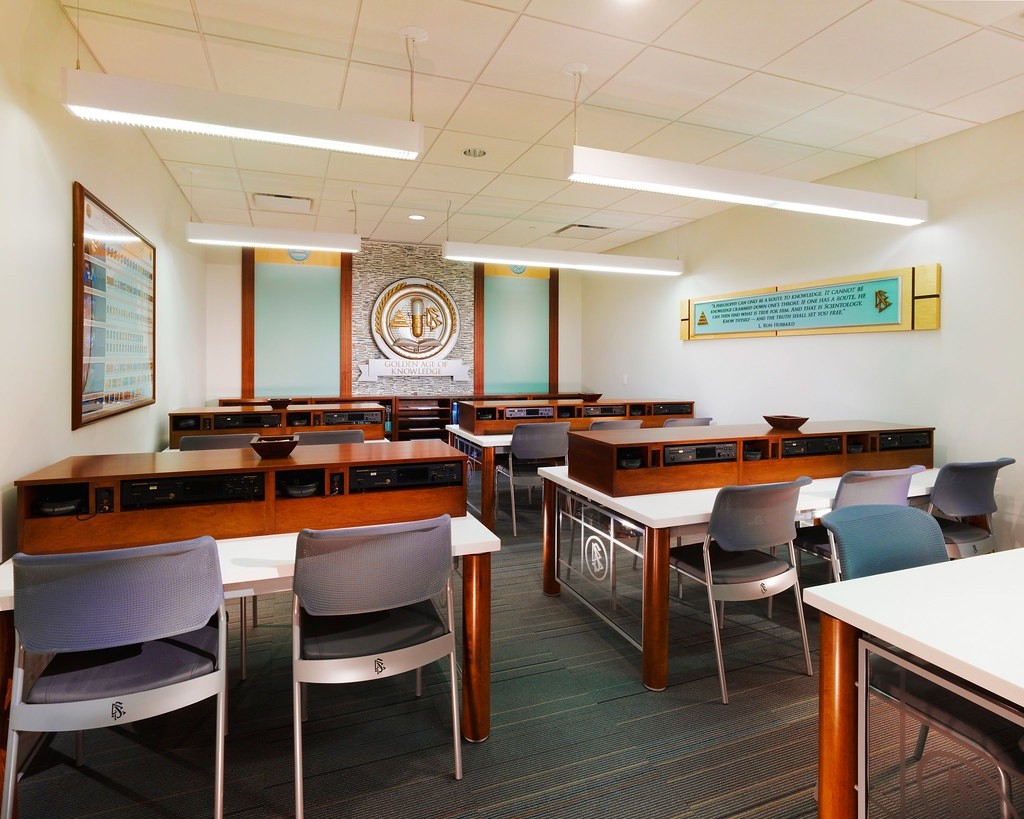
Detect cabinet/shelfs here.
[219,392,603,441]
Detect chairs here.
[495,418,1024,819]
[0,534,229,819]
[294,430,364,445]
[293,513,462,819]
[180,433,259,680]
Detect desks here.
[537,419,935,691]
[802,548,1024,819]
[0,437,501,819]
[445,400,695,533]
[162,403,390,452]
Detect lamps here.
[60,0,425,162]
[185,172,361,253]
[567,71,928,226]
[442,201,684,276]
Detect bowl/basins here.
[250,434,299,459]
[580,394,602,402]
[763,415,809,430]
[268,398,291,409]
